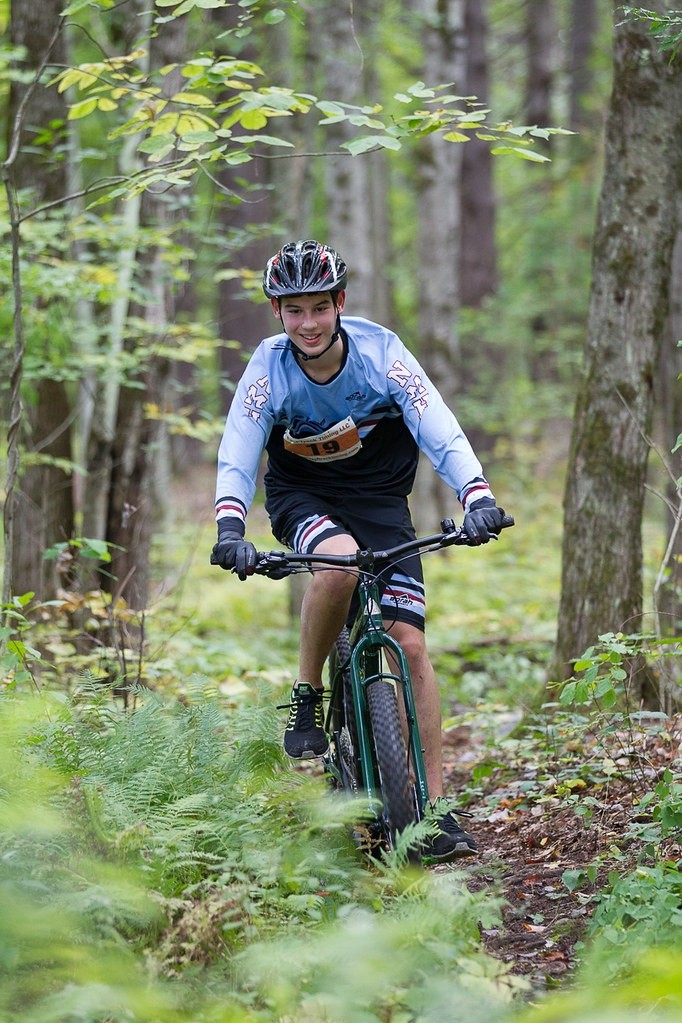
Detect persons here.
[217,239,505,861]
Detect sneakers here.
[420,806,478,862]
[275,679,333,761]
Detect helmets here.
[263,239,347,299]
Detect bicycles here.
[209,516,517,870]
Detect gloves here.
[212,531,256,581]
[463,496,502,546]
